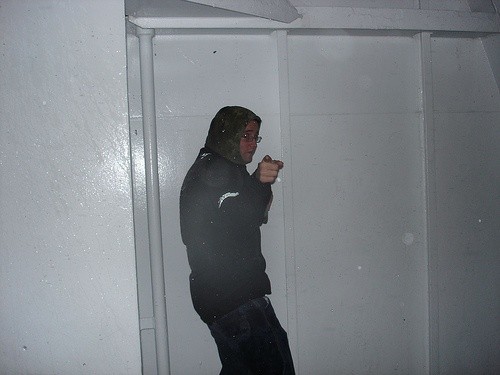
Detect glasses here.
[241,134,262,143]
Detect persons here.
[177,104,290,375]
